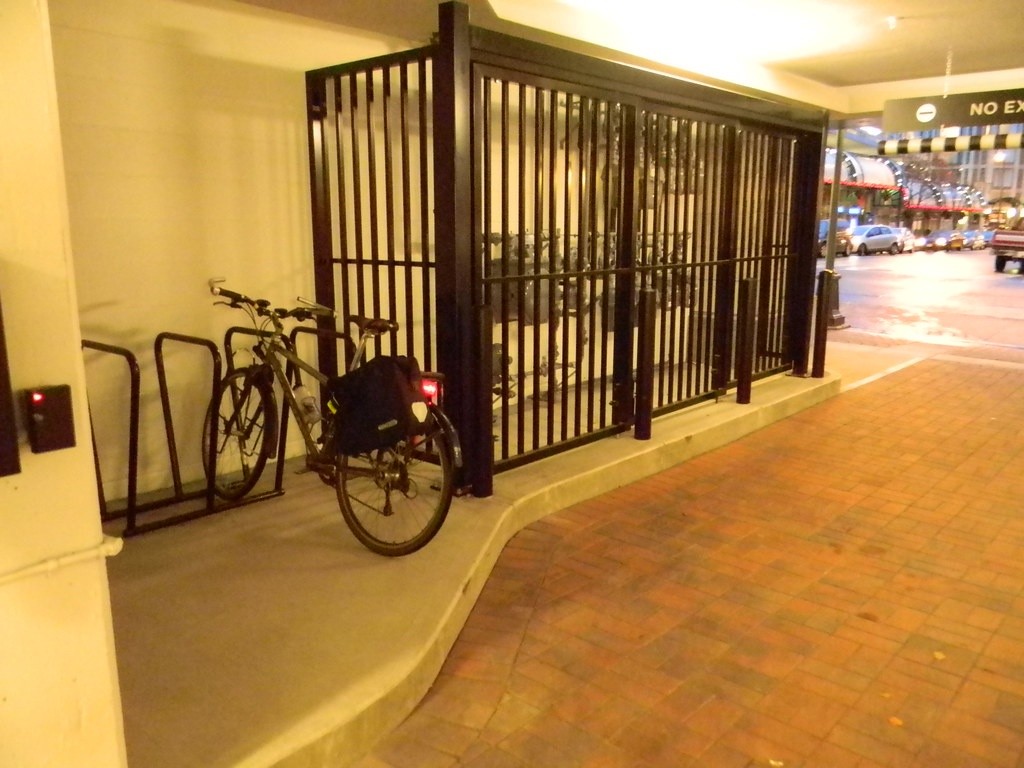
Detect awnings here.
[822,149,903,191]
[902,176,990,214]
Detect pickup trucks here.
[991,216,1024,273]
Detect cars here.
[962,232,985,251]
[851,225,900,255]
[818,220,854,257]
[920,230,966,251]
[893,227,917,254]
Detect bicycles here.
[201,277,462,558]
